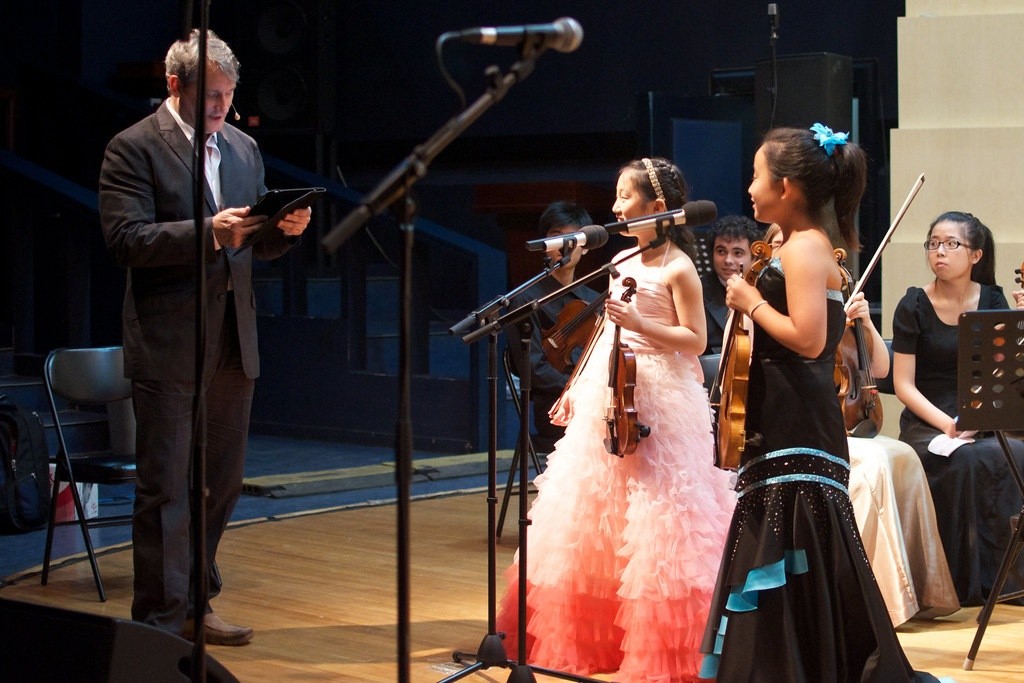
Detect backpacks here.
[0,392,52,536]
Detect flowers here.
[810,122,850,146]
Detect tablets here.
[246,187,326,217]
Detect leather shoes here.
[181,609,254,645]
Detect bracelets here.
[750,300,768,320]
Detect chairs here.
[39,347,223,603]
[496,345,562,541]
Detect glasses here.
[924,240,977,252]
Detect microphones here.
[541,224,609,253]
[459,17,585,54]
[232,103,240,120]
[626,200,718,232]
[767,4,780,38]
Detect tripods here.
[437,233,614,683]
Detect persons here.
[509,199,607,433]
[699,122,945,683]
[495,157,739,683]
[701,214,763,354]
[891,211,1024,607]
[97,28,312,643]
[762,223,961,630]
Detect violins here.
[540,288,620,377]
[951,261,1023,439]
[705,240,774,475]
[605,276,650,461]
[827,245,884,441]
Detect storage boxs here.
[48,463,99,521]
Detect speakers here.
[705,53,889,180]
[0,599,239,683]
